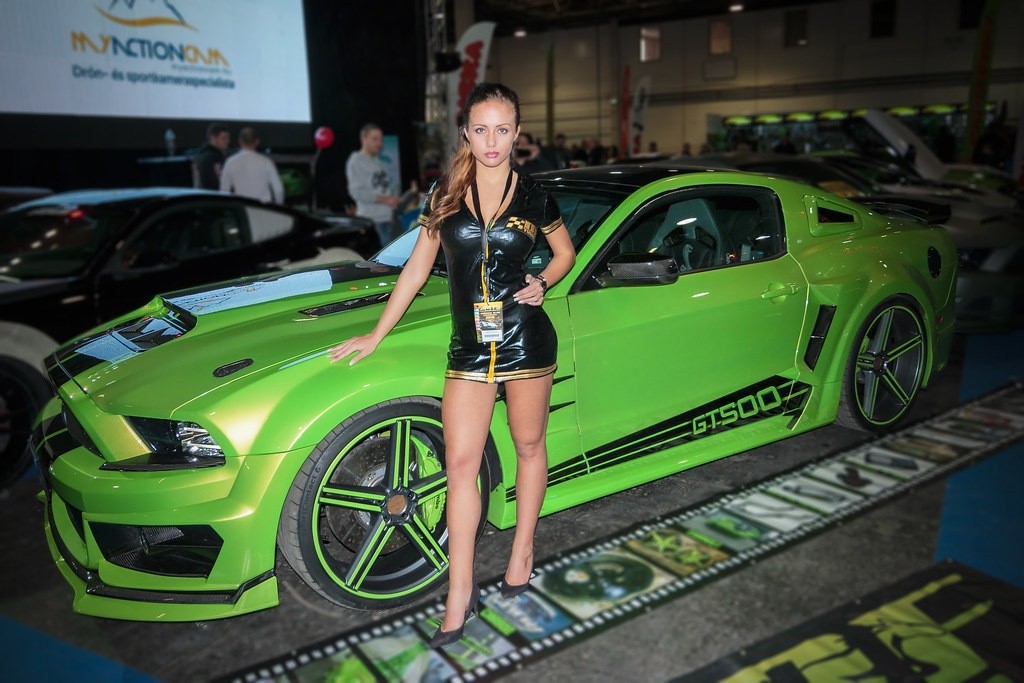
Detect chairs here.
[567,195,733,273]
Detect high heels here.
[500,572,532,601]
[428,580,484,651]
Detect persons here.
[543,126,798,169]
[512,133,555,175]
[192,124,230,190]
[325,82,575,647]
[976,121,1014,170]
[219,127,285,206]
[345,124,401,247]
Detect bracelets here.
[535,275,548,293]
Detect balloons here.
[314,127,334,149]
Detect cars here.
[586,163,1023,247]
[0,187,384,502]
[3,159,960,625]
